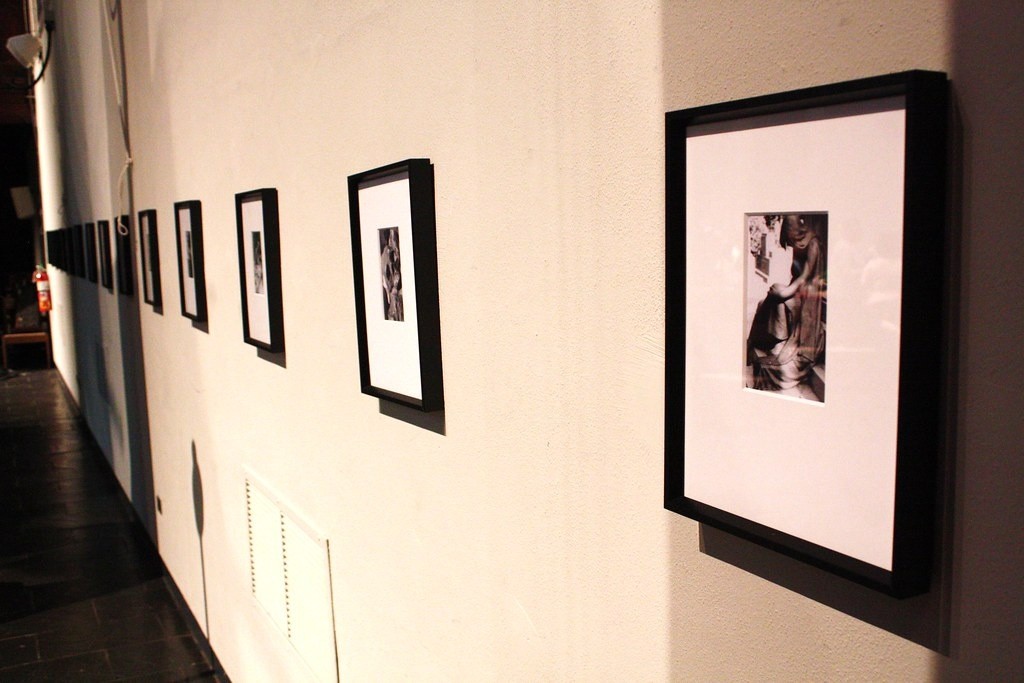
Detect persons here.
[747,214,828,393]
[379,228,404,323]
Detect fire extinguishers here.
[32,265,53,312]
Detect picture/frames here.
[347,157,443,415]
[662,65,951,596]
[174,199,206,322]
[138,209,161,306]
[46,216,133,296]
[234,188,284,353]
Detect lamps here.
[6,20,44,69]
